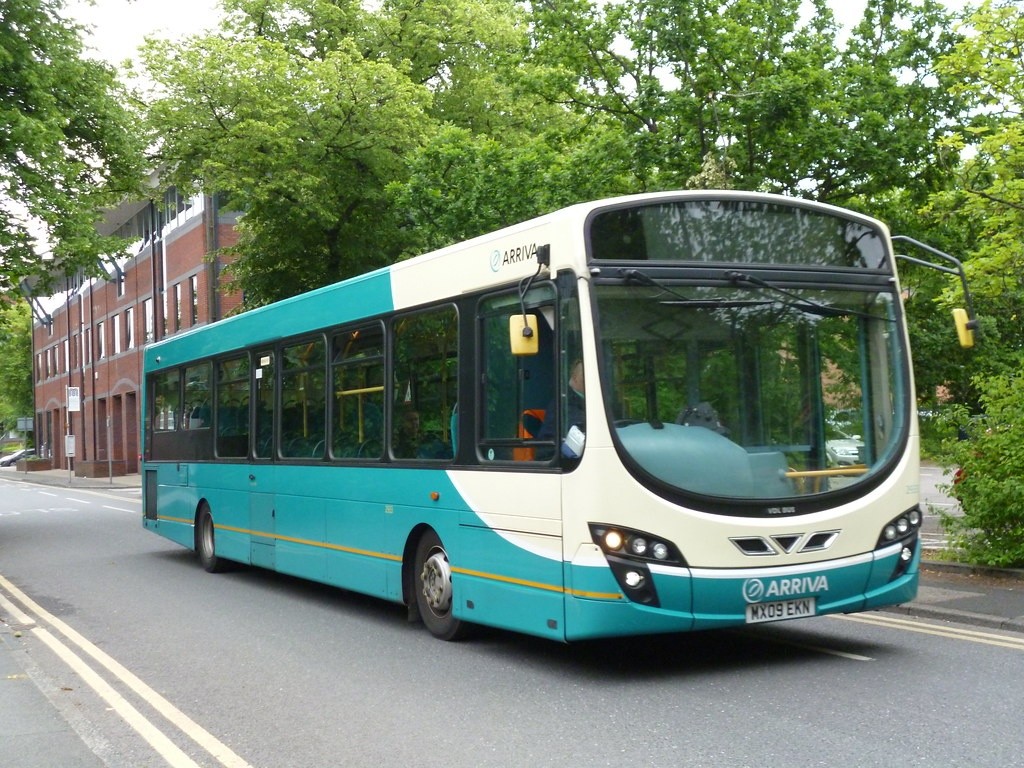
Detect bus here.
[139,188,982,647]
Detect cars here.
[821,429,866,466]
[825,408,862,431]
[0,448,35,467]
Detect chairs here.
[173,397,381,458]
[512,410,545,460]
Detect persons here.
[393,410,443,461]
[539,358,588,466]
